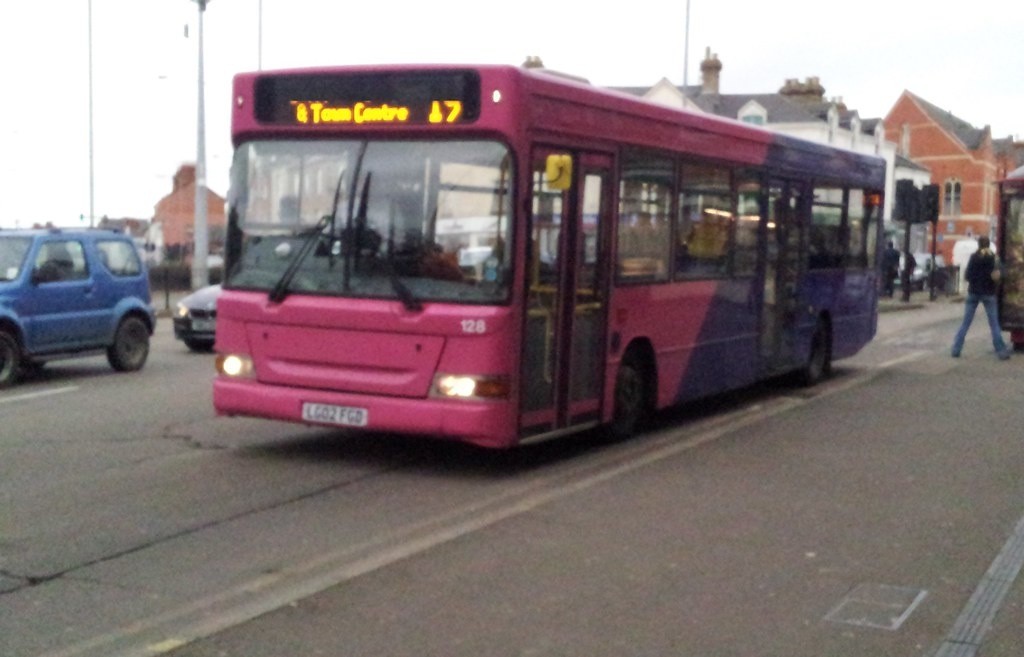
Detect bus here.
[991,165,1024,353]
[209,59,888,455]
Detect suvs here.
[0,227,157,389]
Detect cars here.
[172,282,223,351]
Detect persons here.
[899,247,916,302]
[952,236,1011,360]
[883,242,899,298]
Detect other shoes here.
[999,350,1008,360]
